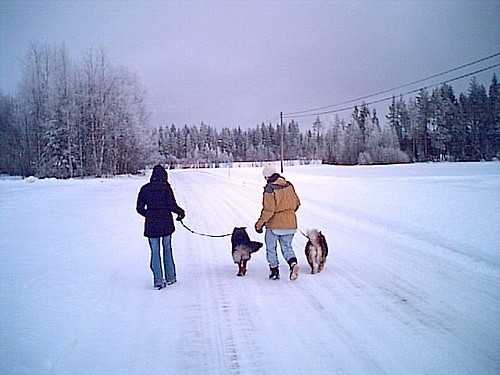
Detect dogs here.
[304,230,329,273]
[232,228,263,277]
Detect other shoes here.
[154,282,166,290]
[269,268,280,280]
[289,262,299,281]
[167,279,176,285]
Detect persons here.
[137,164,185,290]
[255,165,301,281]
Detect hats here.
[263,165,276,176]
[153,165,165,175]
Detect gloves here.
[176,212,185,221]
[255,225,263,233]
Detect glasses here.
[264,176,270,179]
